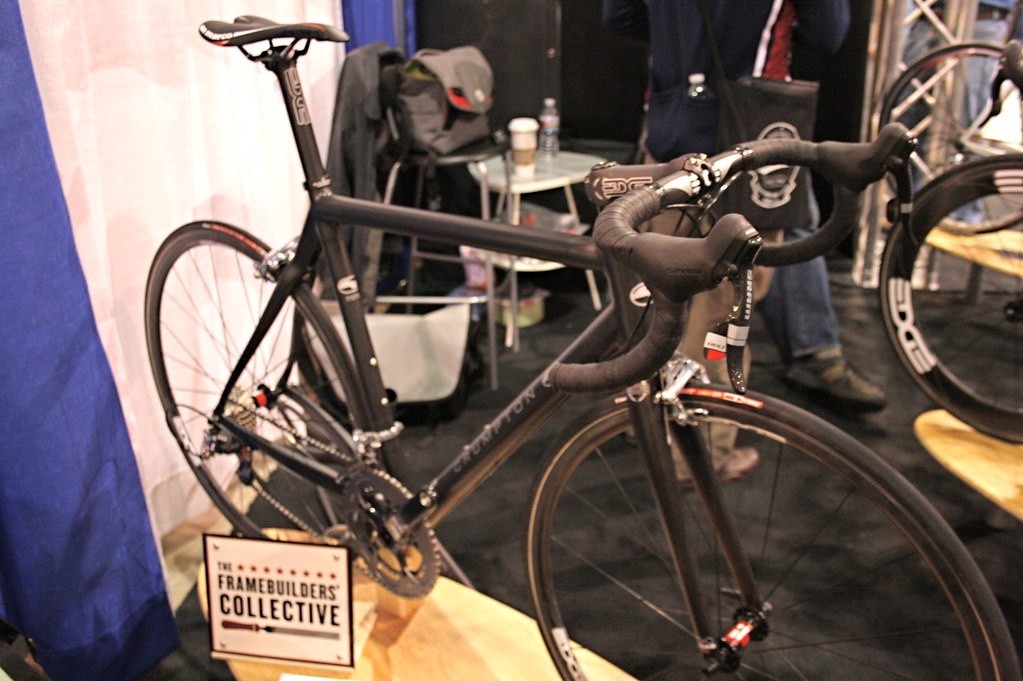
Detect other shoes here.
[786,354,887,407]
[677,446,759,489]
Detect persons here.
[600,0,887,485]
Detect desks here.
[468,149,610,347]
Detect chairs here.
[348,40,517,393]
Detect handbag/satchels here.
[395,47,496,155]
[709,72,820,231]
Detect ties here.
[760,0,794,82]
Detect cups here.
[508,118,540,179]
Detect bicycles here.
[878,0,1023,445]
[140,12,1023,681]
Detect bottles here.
[539,98,560,157]
[687,73,713,102]
